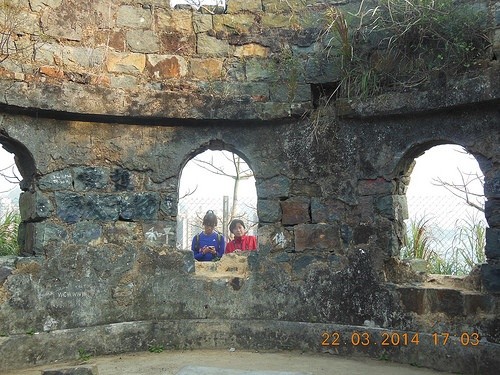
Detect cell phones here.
[205,245,215,249]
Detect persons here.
[190,210,225,262]
[225,219,255,254]
